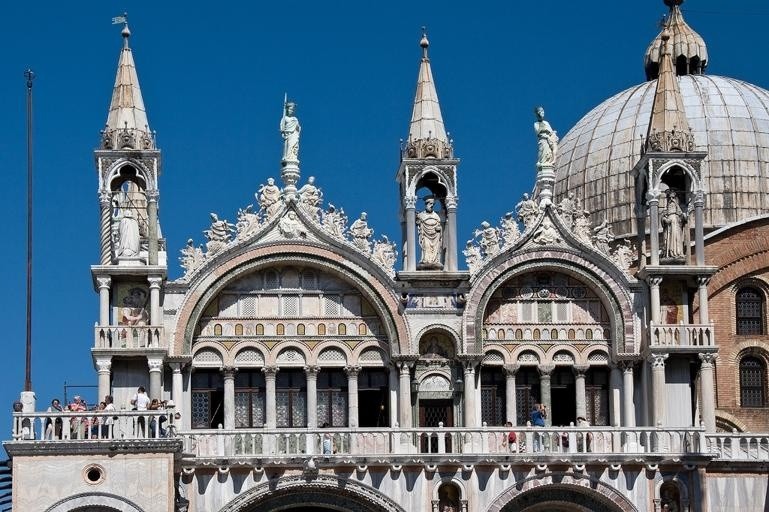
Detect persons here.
[426,336,444,358]
[417,197,442,264]
[464,192,636,269]
[661,289,678,324]
[122,297,149,325]
[661,191,691,257]
[12,385,185,441]
[183,176,398,272]
[320,422,335,454]
[534,106,557,167]
[504,402,595,451]
[280,103,302,161]
